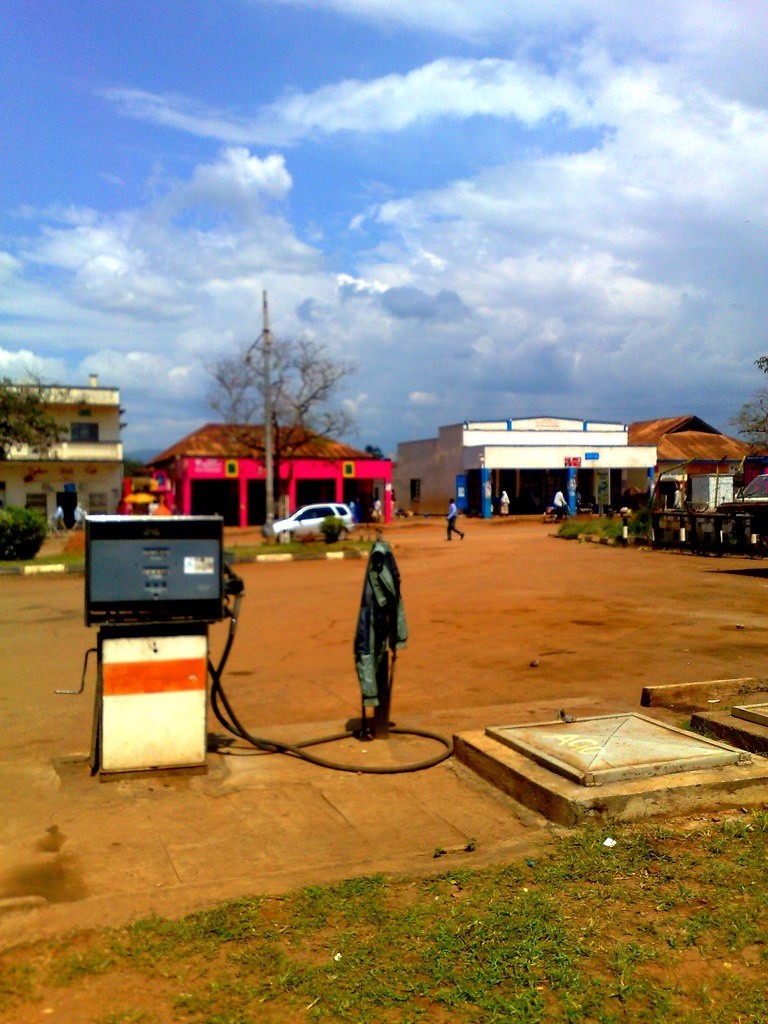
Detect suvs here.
[261,502,358,545]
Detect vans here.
[735,472,768,517]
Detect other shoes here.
[445,537,450,540]
[461,533,464,540]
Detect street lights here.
[265,373,294,517]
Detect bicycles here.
[543,504,570,524]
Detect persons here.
[372,498,382,522]
[553,489,567,524]
[501,491,510,517]
[445,499,464,541]
[72,500,87,531]
[53,503,67,530]
